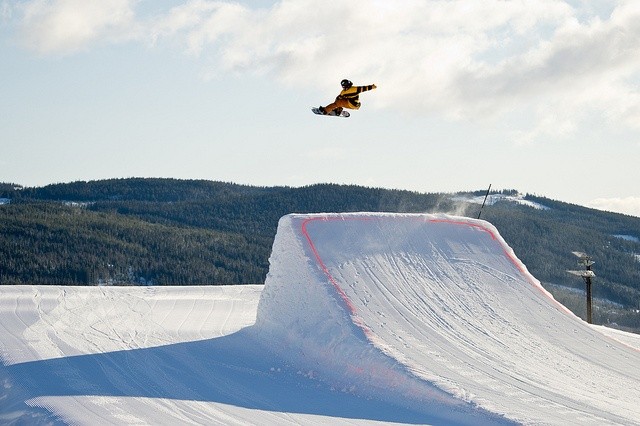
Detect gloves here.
[372,83,376,88]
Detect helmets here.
[341,79,348,85]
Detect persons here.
[319,79,377,116]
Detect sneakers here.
[319,106,327,114]
[335,108,340,115]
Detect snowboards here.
[312,108,350,117]
